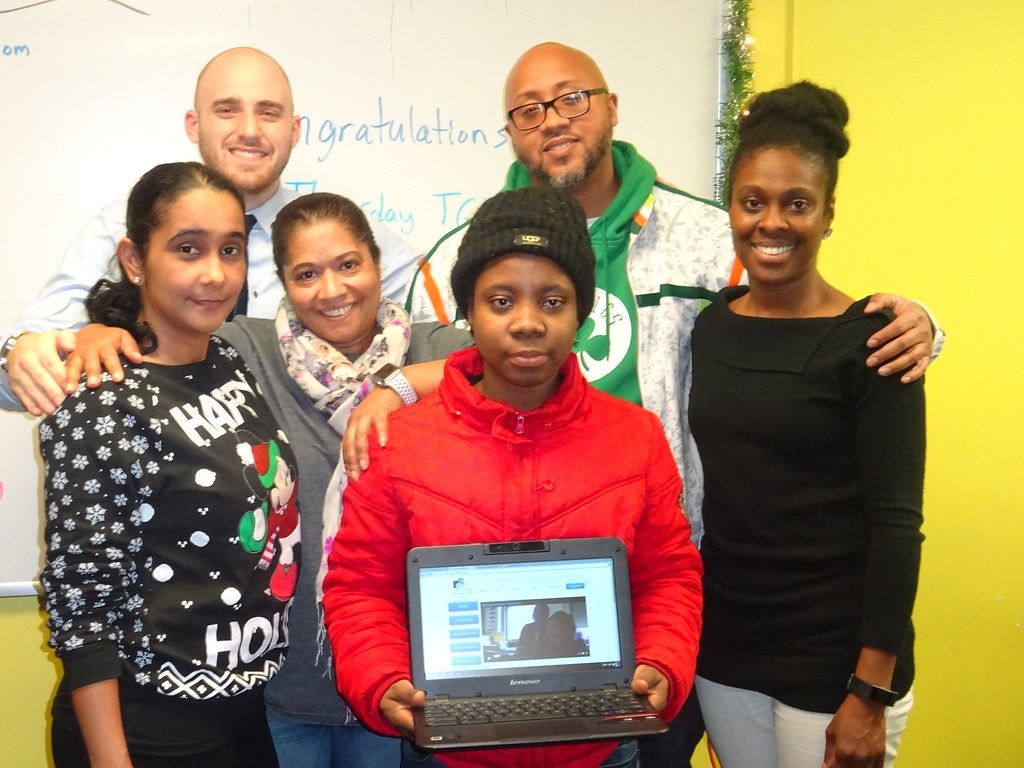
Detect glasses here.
[508,88,609,132]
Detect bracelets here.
[848,675,901,707]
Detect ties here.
[224,214,257,322]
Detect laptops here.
[406,536,671,752]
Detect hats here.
[450,187,596,330]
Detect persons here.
[406,43,948,768]
[37,163,301,768]
[688,82,928,768]
[321,185,704,768]
[1,44,418,416]
[513,602,578,659]
[61,194,478,768]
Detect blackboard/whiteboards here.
[0,0,729,598]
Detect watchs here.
[371,364,418,405]
